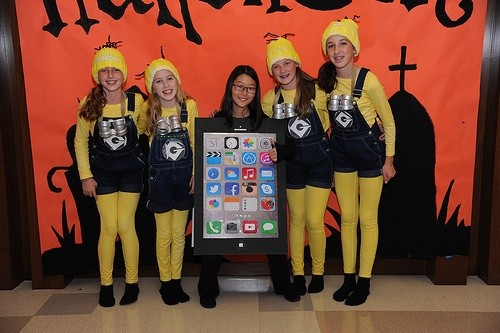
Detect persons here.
[259,38,385,296]
[195,65,300,308]
[317,18,396,306]
[73,46,149,308]
[136,57,199,305]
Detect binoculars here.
[329,95,354,111]
[159,115,181,135]
[274,103,295,119]
[98,119,127,138]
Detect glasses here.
[232,83,257,94]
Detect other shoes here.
[307,275,325,293]
[198,282,216,307]
[333,274,356,303]
[160,282,179,305]
[172,279,190,303]
[99,285,115,307]
[120,286,139,306]
[345,277,370,306]
[292,276,307,296]
[273,276,301,302]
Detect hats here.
[262,31,301,77]
[321,14,361,57]
[91,33,127,85]
[136,44,182,95]
